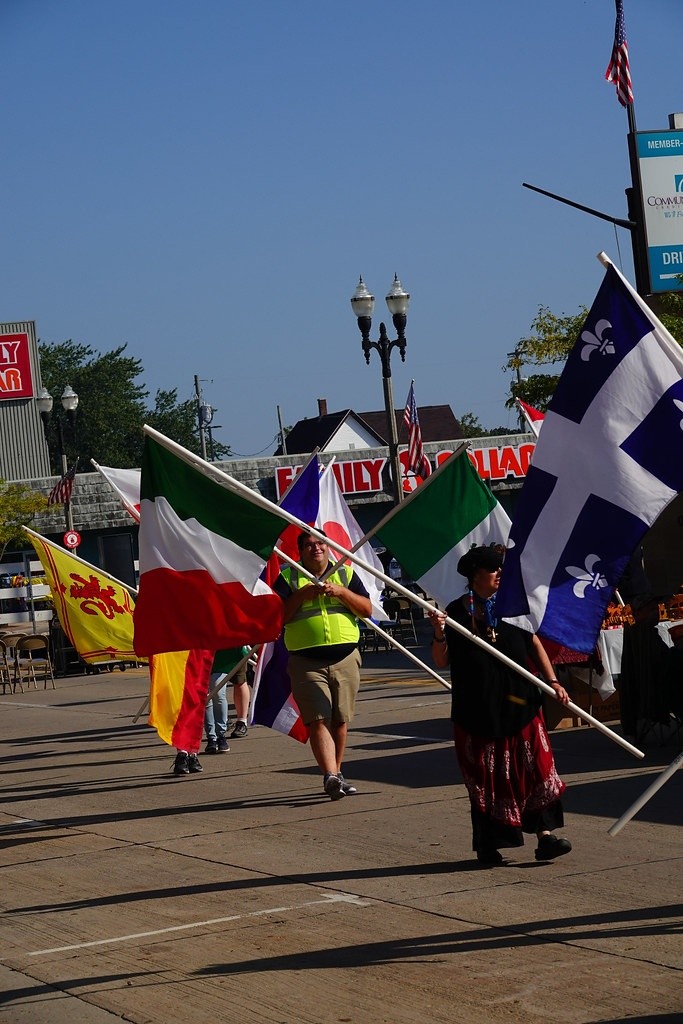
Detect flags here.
[148,649,216,753]
[47,459,77,506]
[605,0,635,107]
[518,399,546,438]
[490,268,683,655]
[402,385,428,481]
[377,451,512,609]
[98,437,390,744]
[25,533,149,664]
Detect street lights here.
[35,380,79,555]
[350,270,412,506]
[507,348,528,434]
[194,374,214,462]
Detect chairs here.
[357,596,419,655]
[0,631,56,695]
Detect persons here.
[273,529,372,800]
[429,544,573,865]
[174,645,255,773]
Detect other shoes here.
[476,848,503,865]
[337,772,358,794]
[323,774,347,802]
[534,834,573,861]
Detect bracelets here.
[433,634,447,643]
[549,680,560,684]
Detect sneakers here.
[219,735,230,753]
[188,753,203,773]
[170,752,191,774]
[231,720,249,739]
[204,739,220,753]
